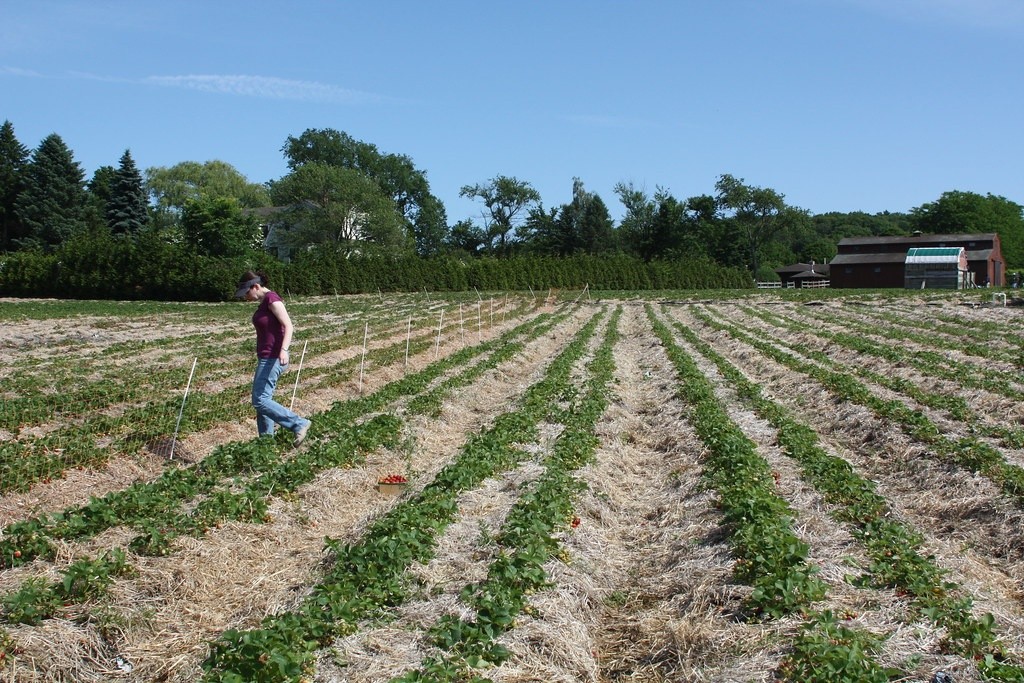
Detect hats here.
[234,276,261,298]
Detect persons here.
[235,269,312,447]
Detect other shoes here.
[293,420,311,448]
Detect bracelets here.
[281,347,288,352]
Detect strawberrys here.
[382,473,407,483]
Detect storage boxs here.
[378,482,407,496]
